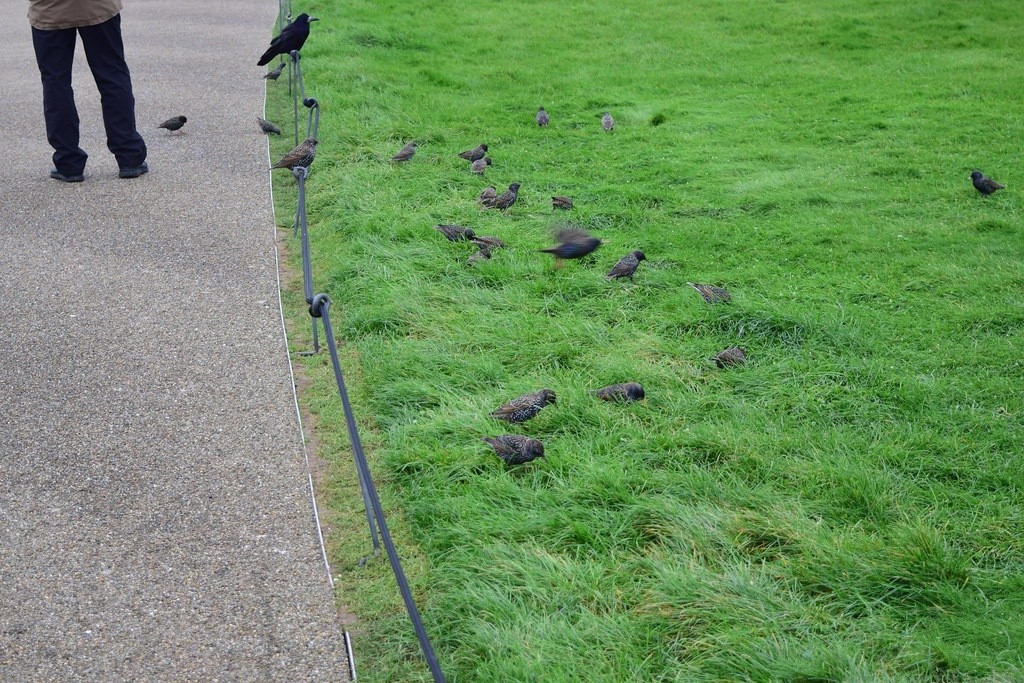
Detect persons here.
[28,0,148,183]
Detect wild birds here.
[458,144,492,175]
[158,116,188,133]
[551,195,575,212]
[255,116,280,135]
[967,171,1008,197]
[257,13,320,83]
[686,281,735,305]
[592,380,646,406]
[393,143,418,162]
[434,222,503,264]
[536,106,548,127]
[601,112,614,130]
[485,433,548,465]
[268,138,318,171]
[488,387,557,424]
[605,250,646,282]
[538,230,611,261]
[477,183,520,212]
[709,343,749,371]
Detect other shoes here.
[119,161,148,177]
[50,167,83,182]
